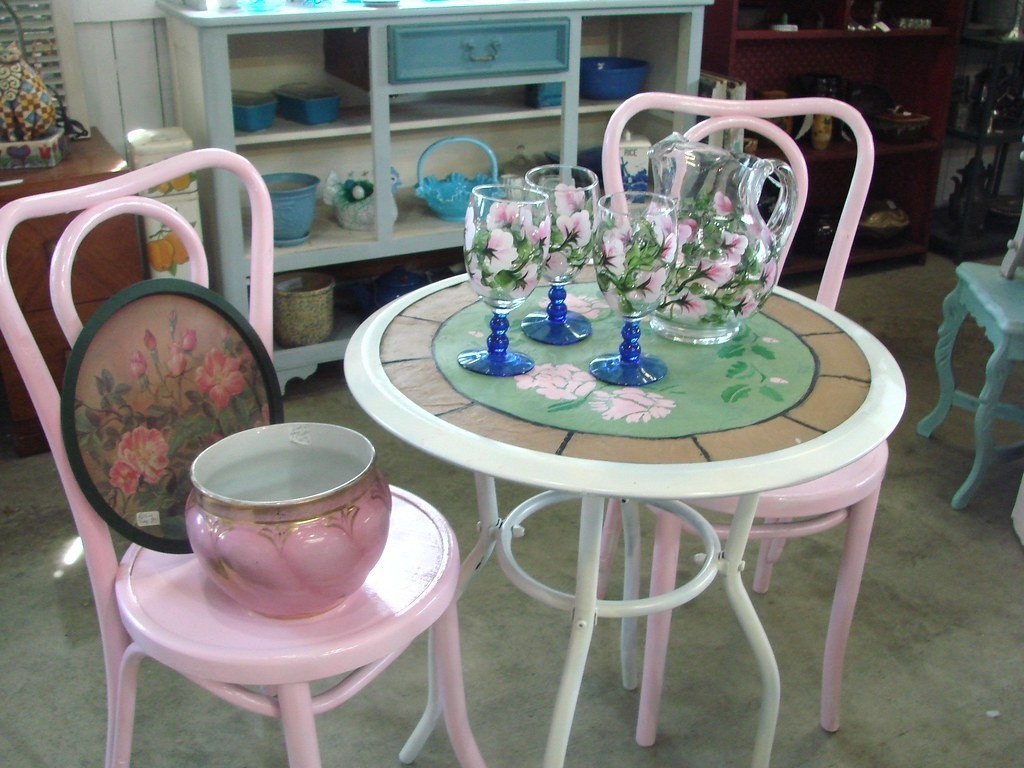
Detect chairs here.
[917,202,1024,511]
[0,146,495,767]
[584,90,890,749]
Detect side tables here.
[343,258,907,768]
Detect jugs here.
[637,131,800,346]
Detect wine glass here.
[521,162,603,346]
[456,183,552,378]
[589,191,681,387]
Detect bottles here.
[812,78,834,149]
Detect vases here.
[260,172,321,248]
[273,271,336,348]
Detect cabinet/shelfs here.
[126,126,208,288]
[929,1,1024,266]
[703,0,966,285]
[156,0,717,401]
[0,126,144,462]
